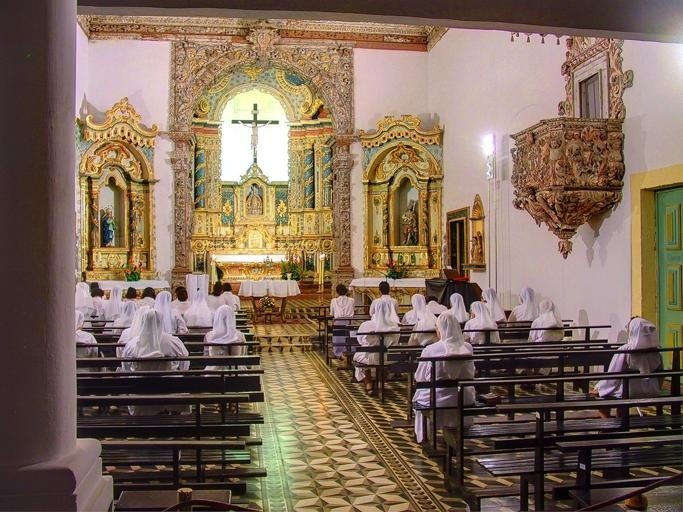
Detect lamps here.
[482,132,496,180]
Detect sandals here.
[365,388,374,395]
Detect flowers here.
[281,259,300,281]
[385,263,405,279]
[123,258,142,281]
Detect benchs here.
[77,313,264,512]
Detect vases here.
[286,273,292,279]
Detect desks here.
[237,280,302,324]
[349,278,426,308]
[85,279,171,298]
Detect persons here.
[411,311,474,443]
[238,122,272,148]
[246,187,262,210]
[590,315,664,419]
[121,308,190,416]
[401,200,418,245]
[101,208,118,247]
[329,280,564,397]
[74,280,249,376]
